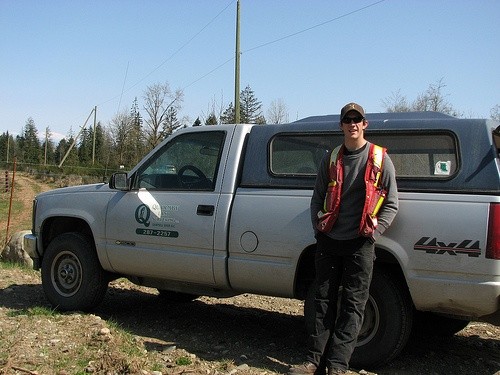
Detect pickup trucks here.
[22,111,500,366]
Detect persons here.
[287,102,399,375]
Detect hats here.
[340,103,365,120]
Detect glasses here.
[342,117,362,124]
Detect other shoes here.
[328,366,344,375]
[287,360,326,375]
